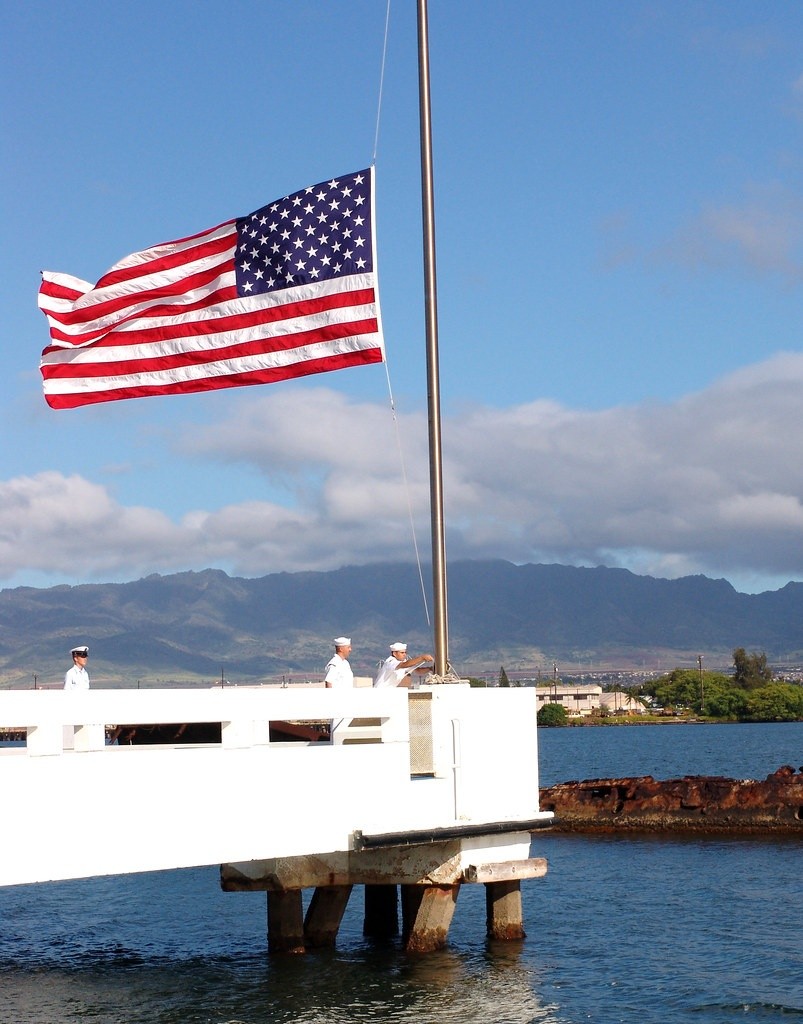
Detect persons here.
[63,647,91,690]
[325,637,354,688]
[373,642,435,687]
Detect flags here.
[38,167,386,410]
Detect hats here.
[69,646,89,657]
[334,637,351,646]
[390,642,407,652]
